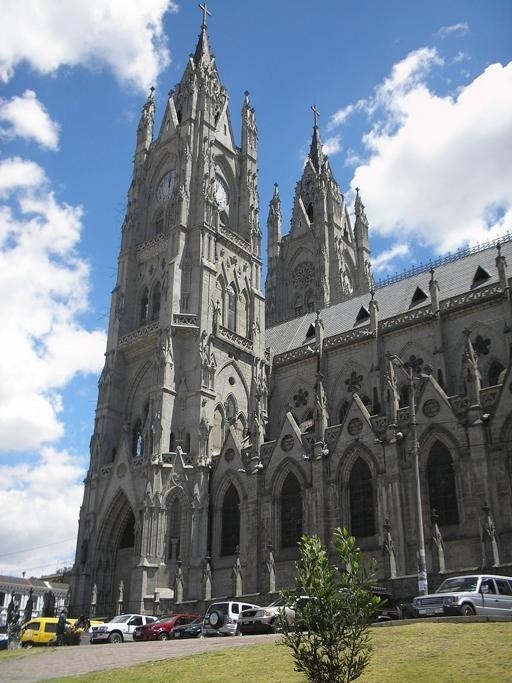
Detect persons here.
[48,608,71,646]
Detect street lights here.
[389,355,428,596]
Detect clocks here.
[155,165,182,205]
